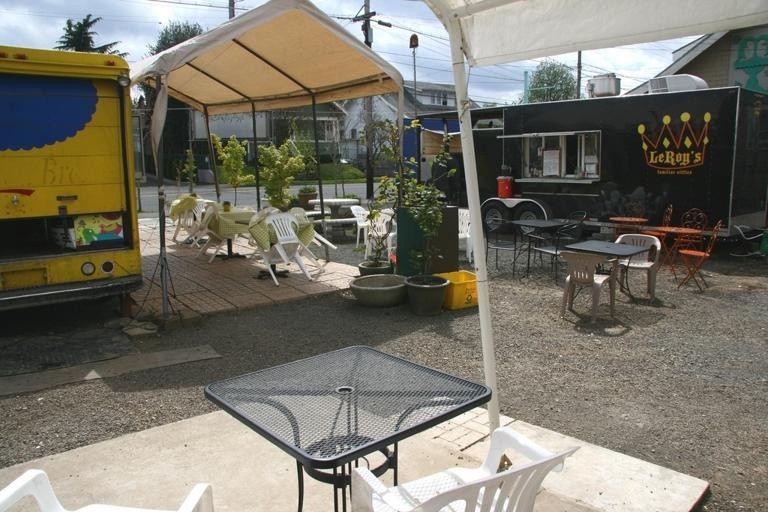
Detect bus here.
[0,47,146,317]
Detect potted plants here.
[351,174,395,276]
[394,157,456,316]
[290,115,317,210]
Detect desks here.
[204,346,492,512]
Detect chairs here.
[482,199,722,324]
[170,193,397,287]
[0,471,214,512]
[458,208,472,264]
[351,426,581,512]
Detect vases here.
[348,275,406,307]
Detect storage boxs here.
[433,270,477,310]
